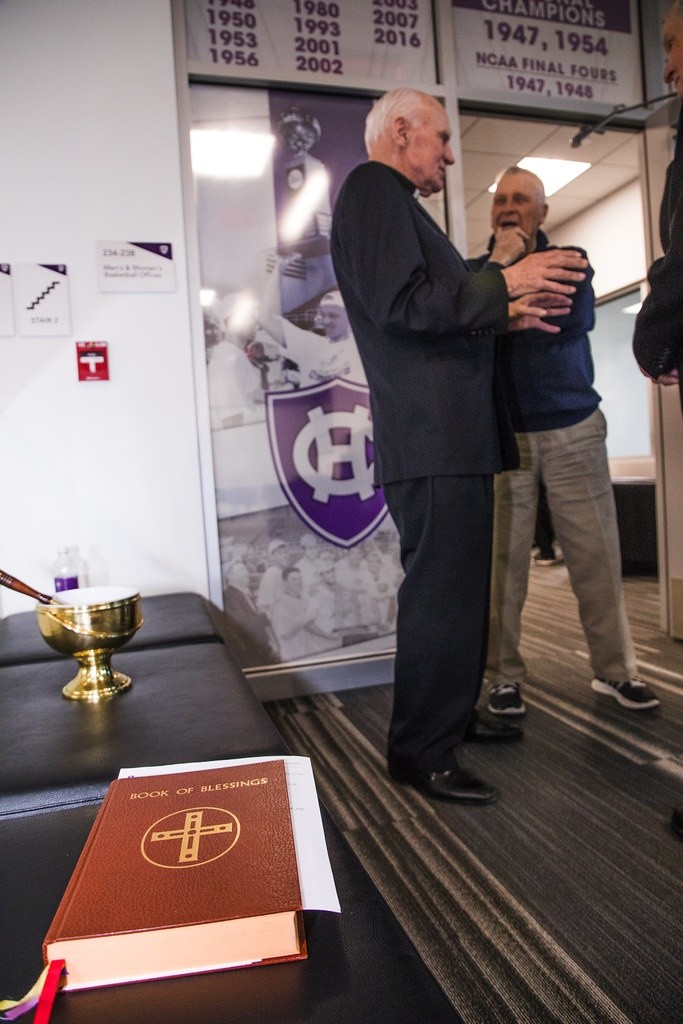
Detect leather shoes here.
[393,711,524,804]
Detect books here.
[40,759,308,997]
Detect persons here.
[631,0,682,414]
[462,163,660,717]
[330,85,589,806]
[204,105,409,664]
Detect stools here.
[0,774,465,1023]
[0,640,294,816]
[0,591,225,665]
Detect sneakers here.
[590,674,662,709]
[486,678,527,714]
